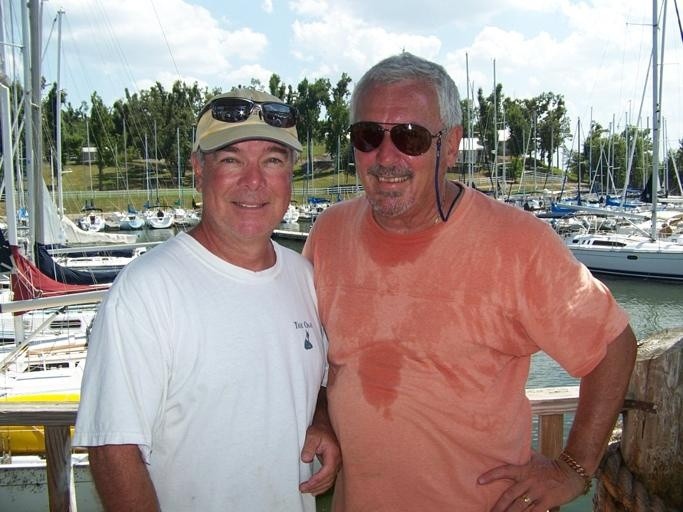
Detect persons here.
[69,87,342,512]
[301,52,637,512]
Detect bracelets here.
[557,451,591,497]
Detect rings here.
[519,493,532,507]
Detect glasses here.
[345,120,447,156]
[193,96,300,143]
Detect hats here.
[192,88,303,158]
